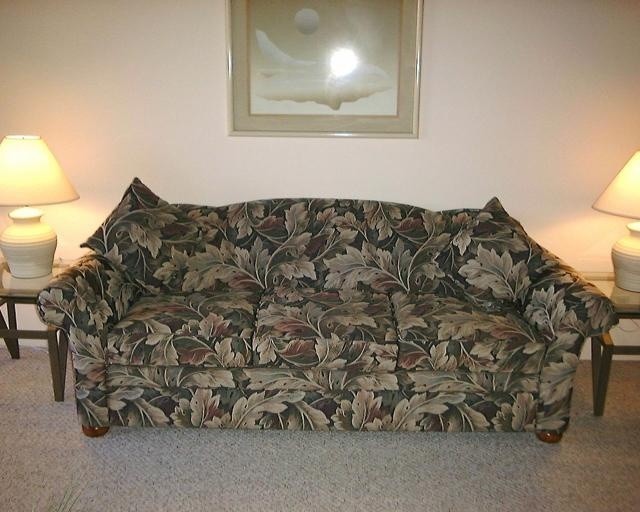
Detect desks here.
[0,264,69,402]
[590,281,639,416]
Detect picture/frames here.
[227,1,426,139]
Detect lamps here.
[593,152,640,296]
[0,135,81,278]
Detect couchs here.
[35,197,616,443]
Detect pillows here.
[79,177,207,298]
[427,196,558,317]
